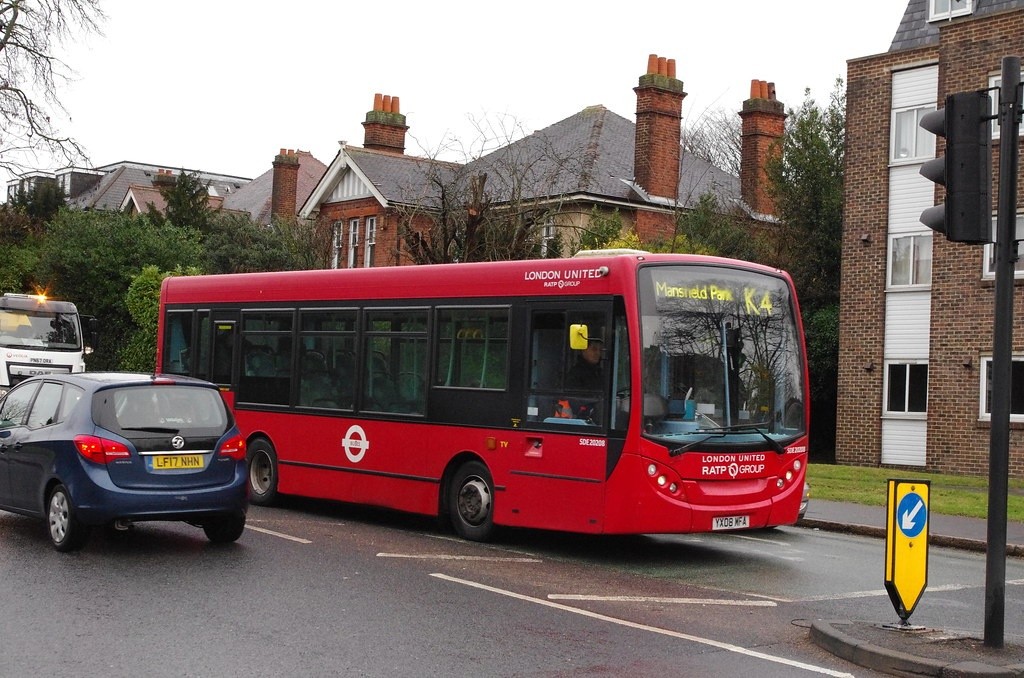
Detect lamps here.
[860,233,873,246]
[964,356,973,367]
[864,362,874,373]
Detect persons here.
[216,324,252,384]
[564,337,630,430]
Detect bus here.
[150,245,814,545]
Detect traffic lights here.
[919,91,993,245]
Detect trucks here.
[0,291,99,399]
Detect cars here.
[0,372,254,552]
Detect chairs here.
[17,325,33,338]
[326,349,355,369]
[362,397,384,412]
[241,345,279,377]
[372,371,395,394]
[372,350,387,375]
[387,399,418,412]
[311,398,344,409]
[307,349,329,376]
